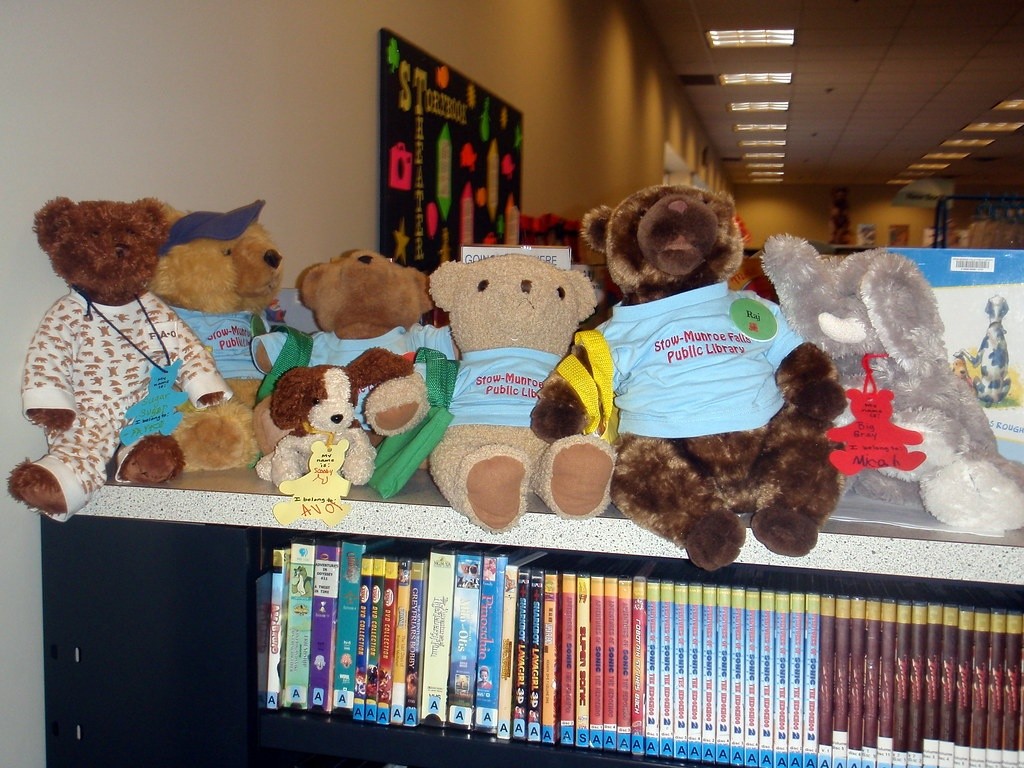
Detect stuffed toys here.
[761,233,1024,533]
[251,249,460,471]
[6,197,235,524]
[149,199,283,472]
[428,254,616,534]
[255,346,415,488]
[529,184,848,571]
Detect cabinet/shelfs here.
[36,466,1024,768]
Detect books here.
[255,536,1024,768]
